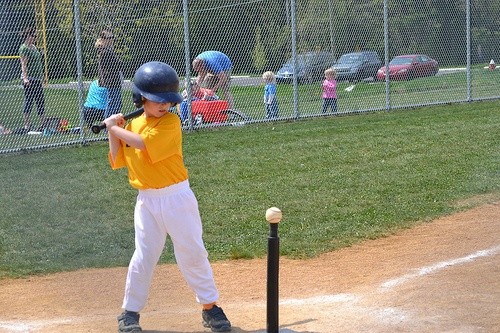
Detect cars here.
[375,54,439,81]
[274,51,336,85]
[325,51,382,83]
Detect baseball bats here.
[91,107,144,134]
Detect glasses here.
[105,36,114,40]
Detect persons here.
[82,78,110,127]
[180,78,221,101]
[93,32,124,136]
[320,68,337,117]
[103,61,231,332]
[260,72,278,119]
[193,52,235,108]
[19,28,46,130]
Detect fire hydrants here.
[488,59,497,70]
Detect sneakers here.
[202,305,231,331]
[118,310,142,333]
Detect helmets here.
[132,61,182,103]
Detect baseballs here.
[265,207,282,224]
